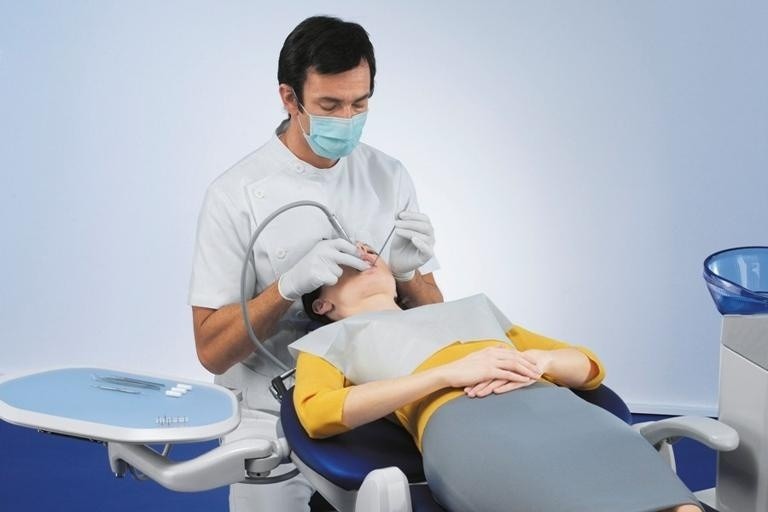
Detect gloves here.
[387,211,436,283]
[278,238,369,302]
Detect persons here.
[283,234,707,511]
[190,13,445,511]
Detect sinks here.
[703,246,768,317]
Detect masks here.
[289,88,369,159]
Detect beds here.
[273,359,739,512]
